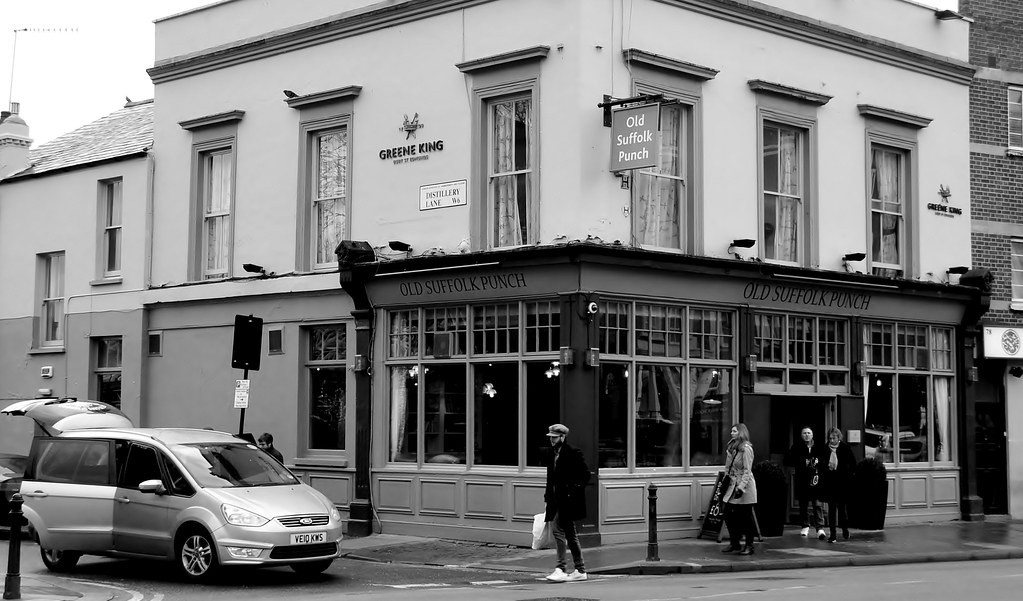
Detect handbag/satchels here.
[720,476,737,503]
[532,500,567,549]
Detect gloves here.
[735,488,743,499]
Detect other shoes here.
[827,537,837,542]
[843,530,849,539]
[737,547,754,555]
[721,544,740,553]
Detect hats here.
[546,423,570,437]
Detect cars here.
[1,396,344,585]
[0,452,30,527]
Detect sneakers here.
[816,530,826,540]
[546,567,568,581]
[800,526,809,536]
[566,569,587,580]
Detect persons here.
[786,427,826,540]
[817,428,856,542]
[258,433,283,465]
[544,424,590,582]
[721,424,758,554]
